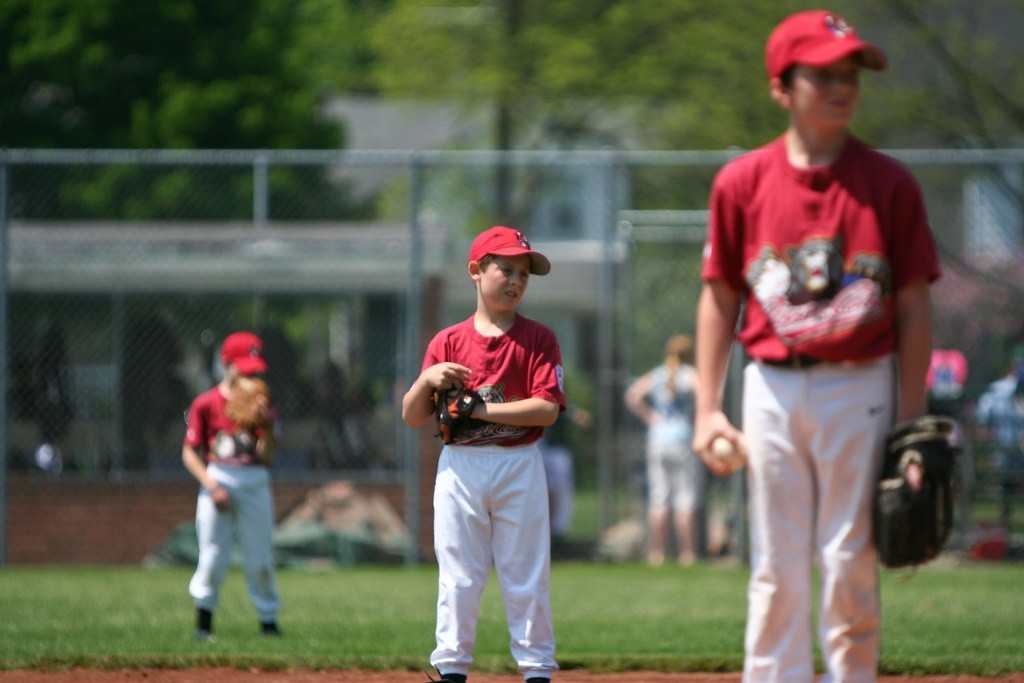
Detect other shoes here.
[423,665,467,683]
[261,621,278,636]
[191,629,214,643]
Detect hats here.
[766,10,888,86]
[221,331,269,374]
[470,226,550,275]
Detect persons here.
[690,8,964,682]
[180,332,282,633]
[972,350,1024,536]
[624,333,698,563]
[402,225,566,683]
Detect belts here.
[765,355,822,368]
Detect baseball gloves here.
[431,376,483,444]
[223,377,276,426]
[869,416,962,569]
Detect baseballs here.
[710,431,747,470]
